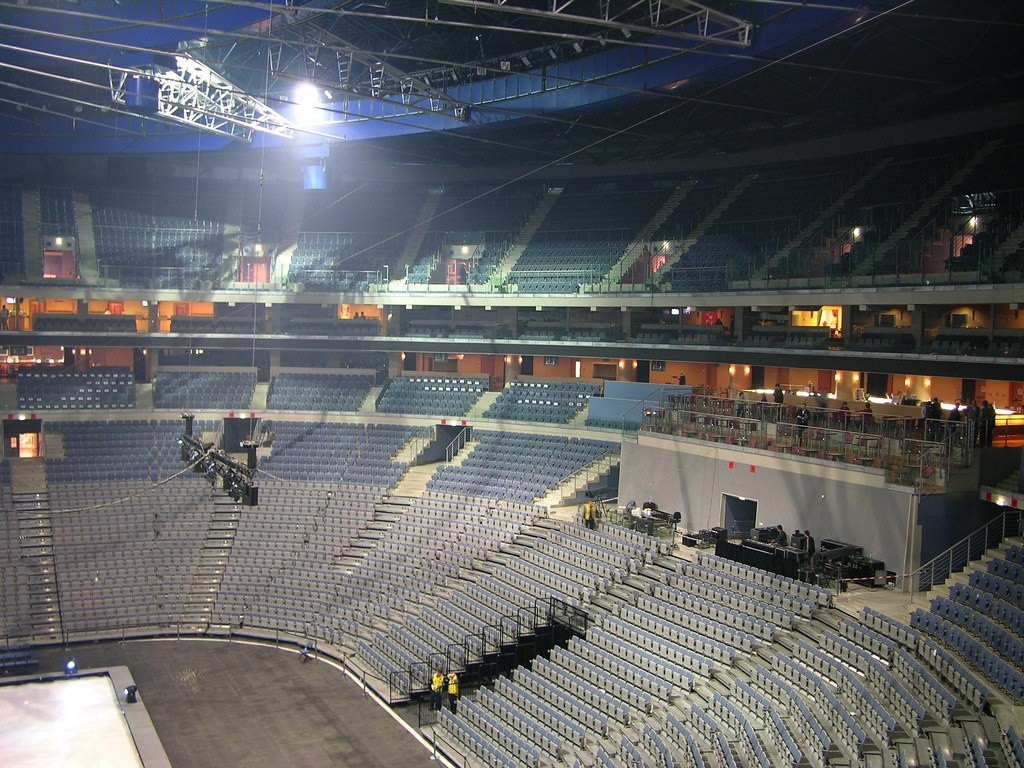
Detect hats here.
[438,670,442,672]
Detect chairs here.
[1,125,1024,294]
[169,314,1024,358]
[111,319,120,331]
[63,319,74,331]
[119,319,127,332]
[95,319,104,332]
[72,319,82,331]
[36,318,46,330]
[46,318,56,331]
[86,319,96,332]
[55,318,64,330]
[103,319,112,332]
[127,318,137,332]
[0,364,1024,768]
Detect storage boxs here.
[787,548,808,564]
[775,545,796,559]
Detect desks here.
[717,418,727,434]
[817,432,837,450]
[904,464,920,487]
[917,444,939,462]
[857,457,874,466]
[714,435,725,442]
[715,538,798,581]
[776,444,791,453]
[818,410,837,428]
[645,425,655,431]
[850,414,861,432]
[738,402,757,417]
[877,417,900,437]
[804,448,817,457]
[829,452,845,462]
[766,405,789,422]
[797,429,807,447]
[735,438,749,446]
[901,416,925,430]
[738,421,750,438]
[709,400,728,414]
[694,416,702,428]
[856,437,877,458]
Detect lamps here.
[477,68,487,76]
[573,42,583,53]
[597,35,606,46]
[450,70,461,83]
[520,56,534,69]
[500,60,511,71]
[423,76,432,87]
[64,656,80,674]
[620,28,632,38]
[124,686,138,704]
[547,48,558,59]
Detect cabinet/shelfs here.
[850,557,885,588]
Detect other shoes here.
[429,708,434,710]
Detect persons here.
[773,384,784,403]
[838,401,873,430]
[583,500,596,530]
[352,311,366,319]
[714,314,734,337]
[678,371,686,385]
[918,396,996,446]
[0,304,10,331]
[736,391,747,418]
[759,393,767,403]
[804,529,816,565]
[795,402,811,447]
[771,524,787,546]
[428,669,446,714]
[447,673,459,714]
